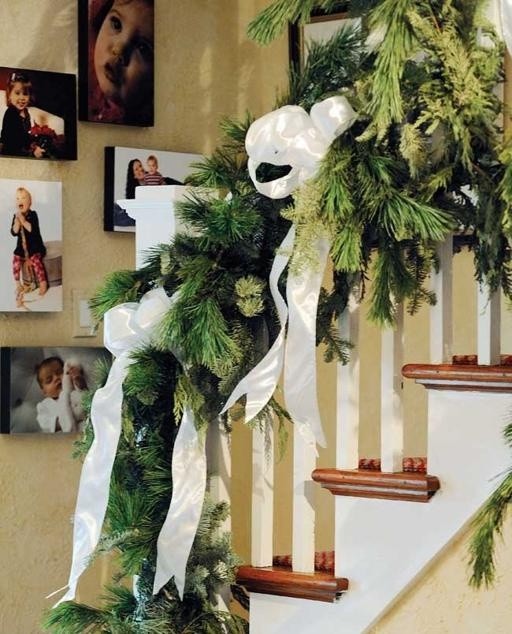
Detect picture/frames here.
[288,0,376,106]
[73,290,100,337]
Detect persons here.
[9,186,48,295]
[89,0,154,126]
[143,154,163,185]
[1,71,63,161]
[125,158,184,200]
[35,356,88,433]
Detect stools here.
[21,240,62,288]
